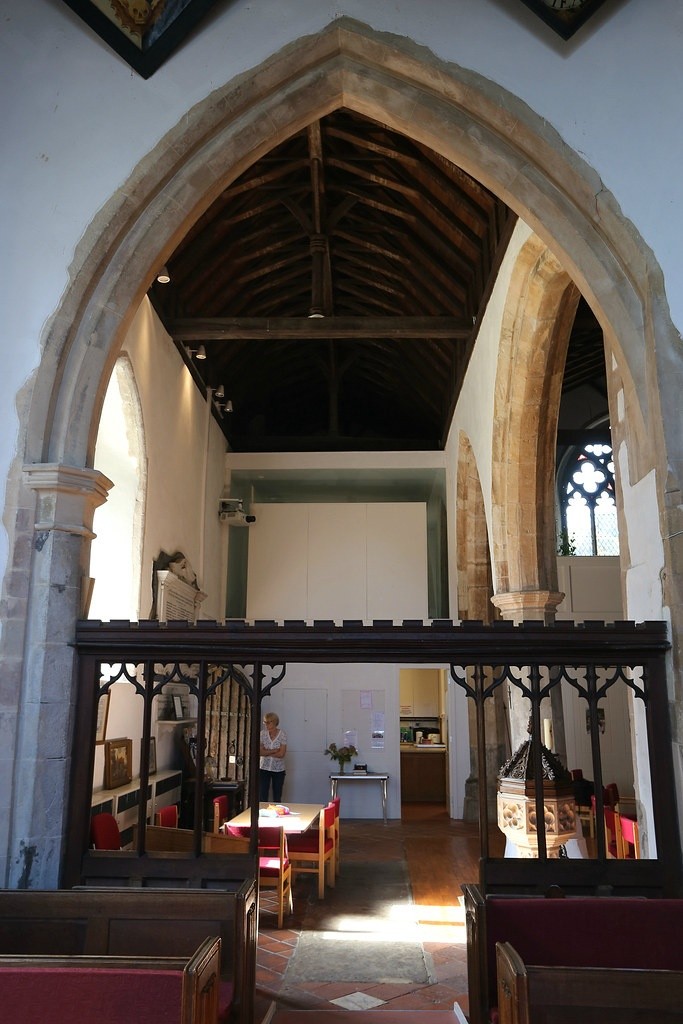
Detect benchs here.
[459,880,683,1024]
[0,934,222,1024]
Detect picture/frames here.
[140,737,157,776]
[95,690,111,744]
[105,737,131,789]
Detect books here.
[352,763,368,776]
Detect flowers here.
[324,743,359,764]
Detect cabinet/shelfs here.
[92,769,185,834]
[403,752,446,802]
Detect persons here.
[260,712,287,803]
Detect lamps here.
[219,400,233,412]
[187,345,206,359]
[210,384,224,398]
[157,265,170,284]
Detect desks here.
[218,802,325,912]
[330,771,389,829]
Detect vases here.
[340,760,344,774]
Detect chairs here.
[93,794,341,929]
[182,743,240,820]
